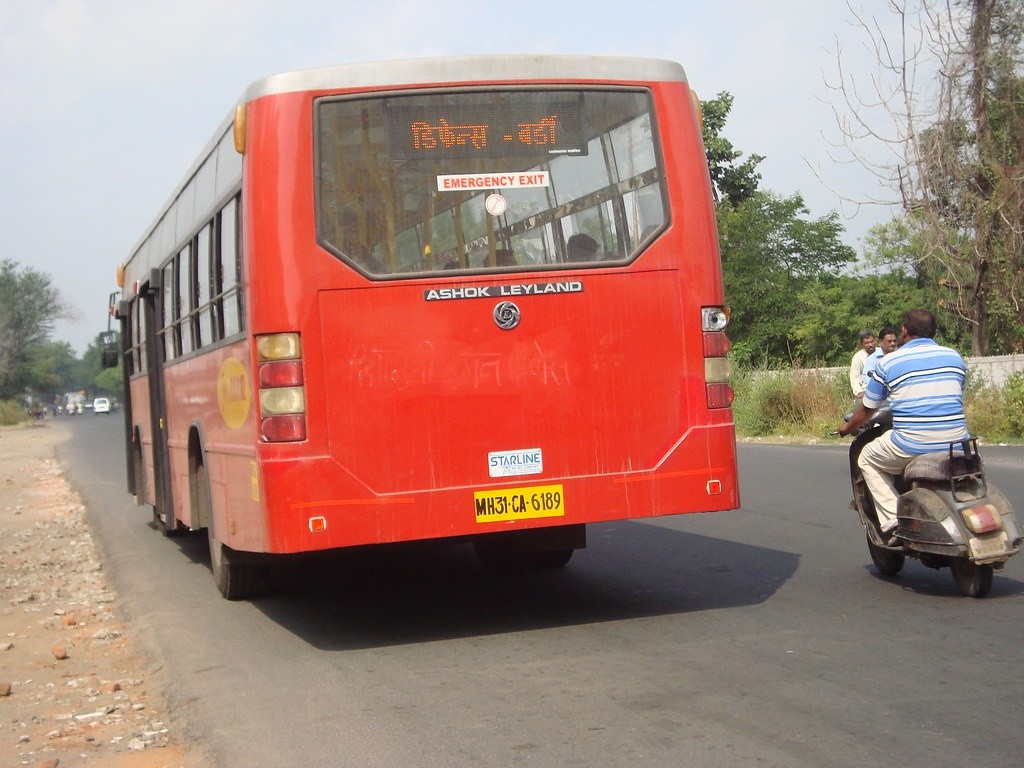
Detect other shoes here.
[887,526,903,547]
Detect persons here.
[29,401,84,420]
[567,234,600,262]
[838,309,970,547]
[483,249,518,267]
[849,328,898,414]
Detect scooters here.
[828,398,1024,599]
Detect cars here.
[91,397,111,416]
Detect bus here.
[98,53,742,601]
